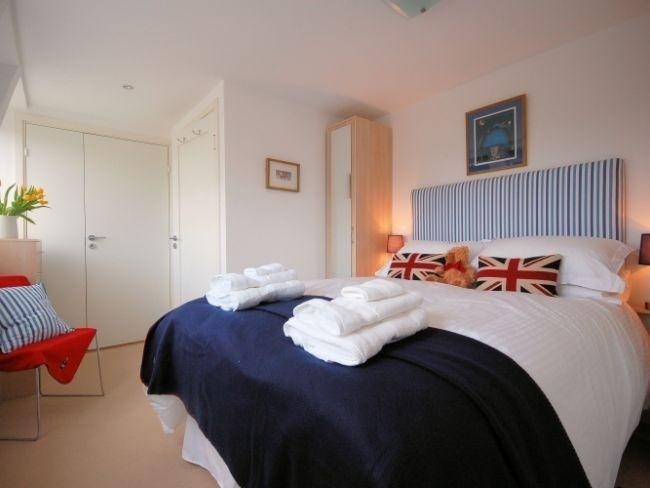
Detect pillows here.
[0,283,75,356]
[477,254,562,298]
[387,252,446,283]
[469,237,633,296]
[374,239,492,281]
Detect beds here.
[137,157,650,488]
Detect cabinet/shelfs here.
[324,113,392,277]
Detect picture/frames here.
[266,158,300,192]
[465,94,528,176]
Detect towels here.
[283,279,429,370]
[206,263,307,311]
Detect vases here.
[0,215,18,239]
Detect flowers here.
[0,177,50,225]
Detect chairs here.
[0,274,105,442]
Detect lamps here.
[387,234,404,255]
[637,231,650,265]
